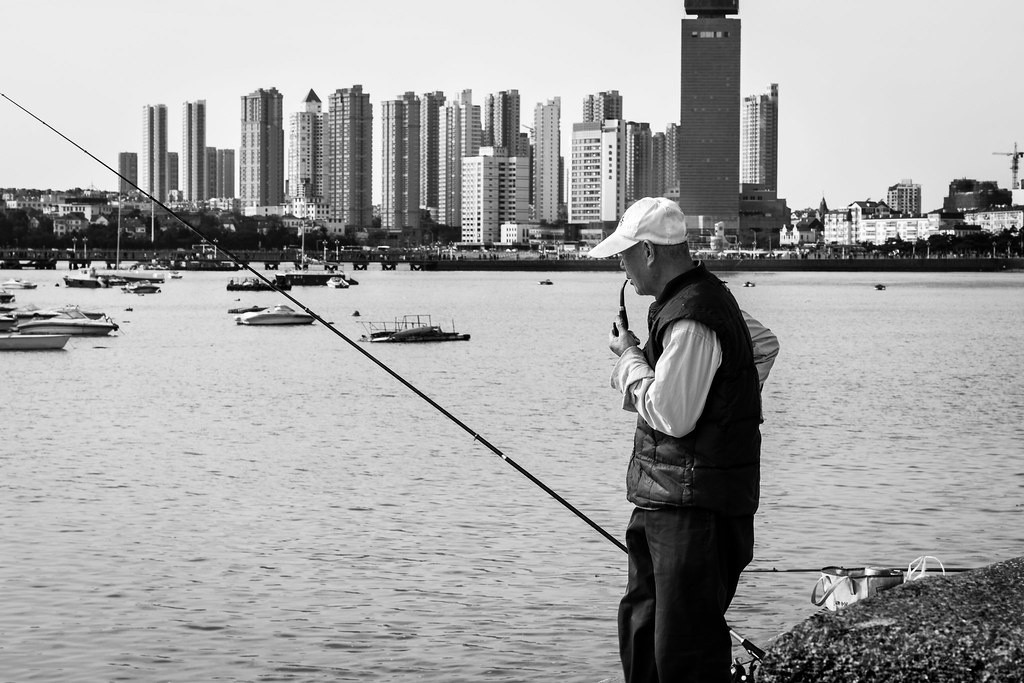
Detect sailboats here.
[285,220,346,287]
[90,196,165,286]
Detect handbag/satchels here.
[811,566,904,612]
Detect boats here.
[356,315,471,343]
[63,275,110,288]
[0,316,18,332]
[0,334,72,349]
[0,290,15,303]
[226,274,293,292]
[325,277,350,289]
[2,279,37,290]
[14,304,106,323]
[17,310,119,336]
[121,280,160,294]
[235,305,319,327]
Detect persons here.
[587,197,780,683]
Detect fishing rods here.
[741,564,974,574]
[0,88,770,658]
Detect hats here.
[586,198,689,258]
